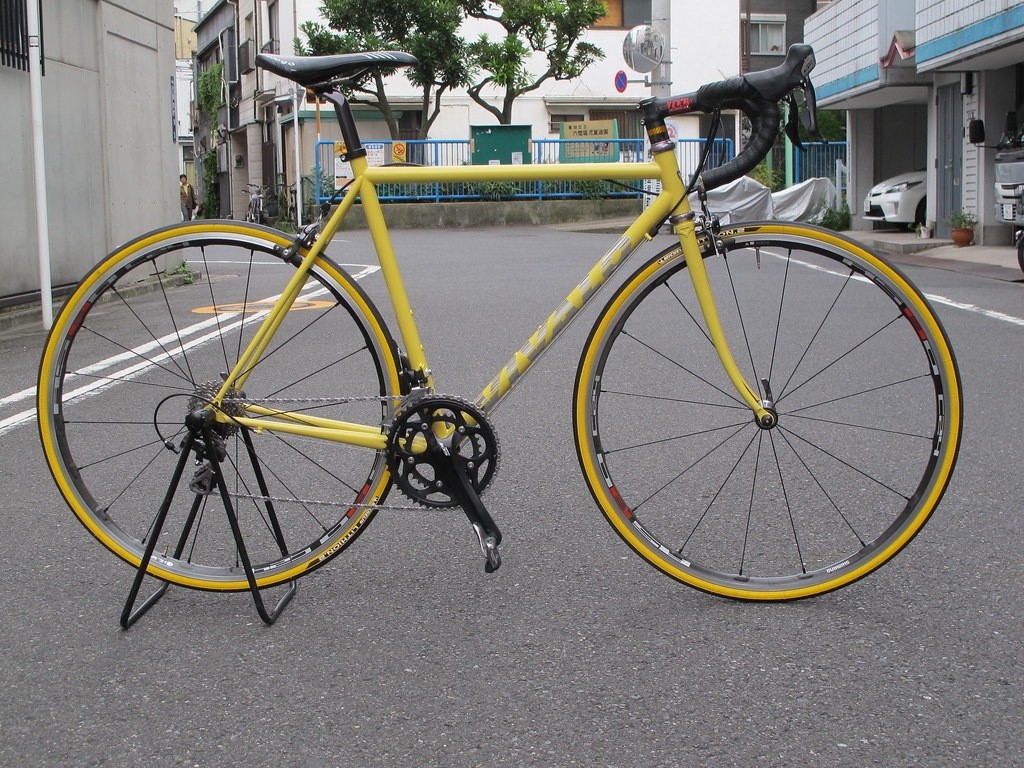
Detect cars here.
[862,162,927,229]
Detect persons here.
[180,174,200,221]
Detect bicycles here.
[37,43,964,629]
[241,184,271,224]
[278,182,298,227]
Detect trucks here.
[969,104,1024,272]
